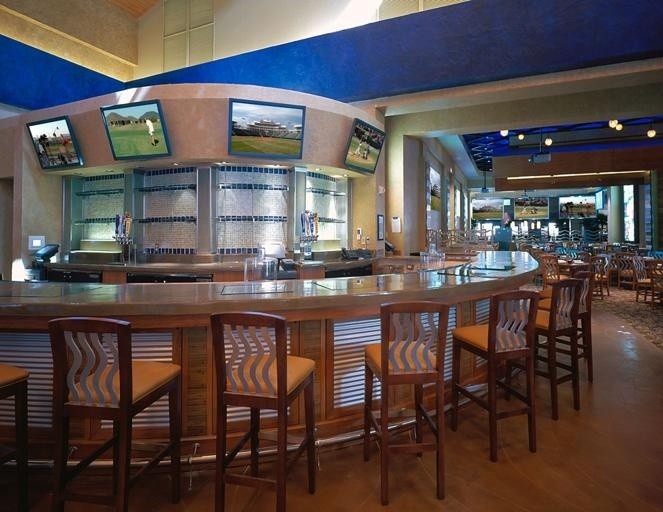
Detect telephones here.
[340,247,359,261]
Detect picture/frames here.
[378,214,384,240]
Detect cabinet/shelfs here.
[371,254,465,275]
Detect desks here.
[0,251,539,476]
[558,258,589,275]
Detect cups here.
[433,252,445,273]
[259,257,278,291]
[243,256,261,287]
[418,251,430,273]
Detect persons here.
[530,206,537,214]
[38,138,48,157]
[521,204,528,214]
[60,133,74,162]
[562,202,570,217]
[579,201,584,217]
[365,144,370,160]
[354,141,362,156]
[586,203,591,217]
[363,148,366,159]
[144,116,156,146]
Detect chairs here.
[512,235,645,252]
[531,248,545,286]
[539,252,569,291]
[594,254,611,296]
[539,264,593,299]
[632,255,652,302]
[539,270,596,382]
[364,300,450,507]
[648,258,663,309]
[590,257,605,301]
[506,277,584,421]
[211,311,317,512]
[0,362,31,512]
[615,252,635,289]
[450,290,540,462]
[47,316,181,512]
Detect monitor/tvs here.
[99,99,172,160]
[259,241,285,259]
[514,196,549,220]
[558,195,597,220]
[343,117,386,175]
[30,244,60,259]
[472,197,505,221]
[228,97,305,161]
[24,115,84,172]
